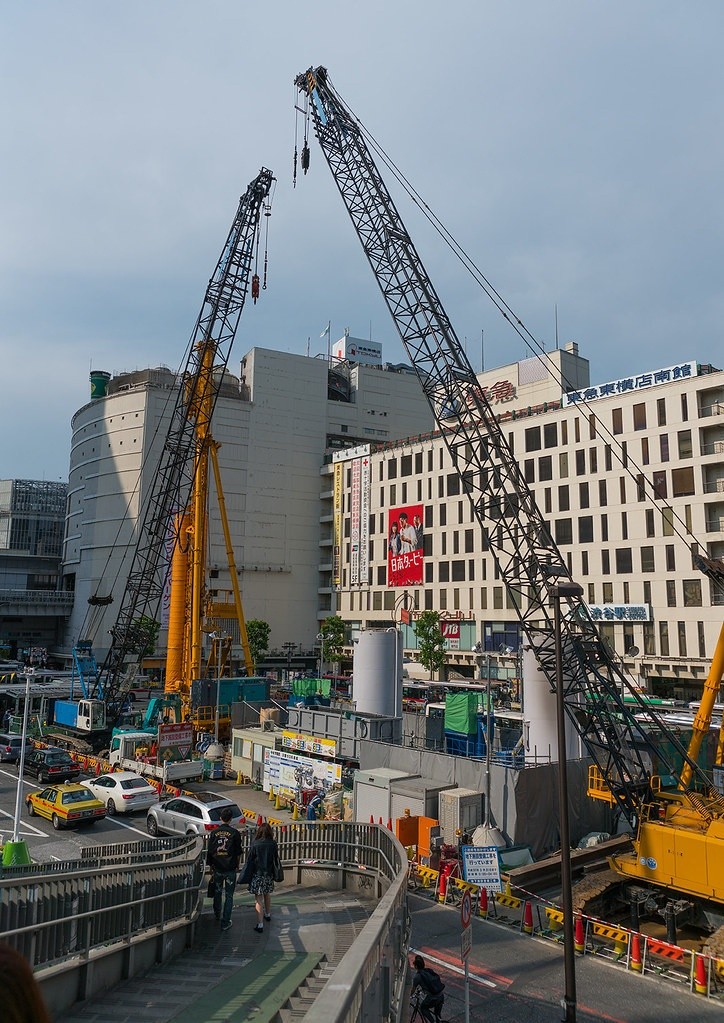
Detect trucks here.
[107,734,206,786]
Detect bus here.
[426,692,723,745]
[323,669,501,712]
[0,646,151,730]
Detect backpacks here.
[417,967,445,996]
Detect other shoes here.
[264,913,271,921]
[254,923,263,933]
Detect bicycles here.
[410,986,448,1023]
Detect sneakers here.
[221,920,232,930]
[213,911,220,919]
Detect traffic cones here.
[523,902,533,932]
[368,814,393,838]
[437,873,449,903]
[291,805,300,821]
[631,935,642,971]
[268,785,282,810]
[574,917,585,952]
[695,956,708,995]
[235,772,243,785]
[478,888,489,917]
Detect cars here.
[14,749,80,783]
[25,781,106,831]
[81,771,160,816]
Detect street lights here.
[11,647,49,842]
[316,633,335,678]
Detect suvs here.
[0,734,33,761]
[145,790,245,841]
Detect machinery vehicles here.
[98,694,185,761]
[48,165,278,755]
[295,65,724,985]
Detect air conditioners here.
[262,720,275,732]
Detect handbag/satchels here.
[237,864,251,884]
[274,868,284,882]
[207,876,215,898]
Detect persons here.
[411,956,445,1023]
[306,793,326,830]
[206,811,243,930]
[389,513,423,557]
[245,823,284,934]
